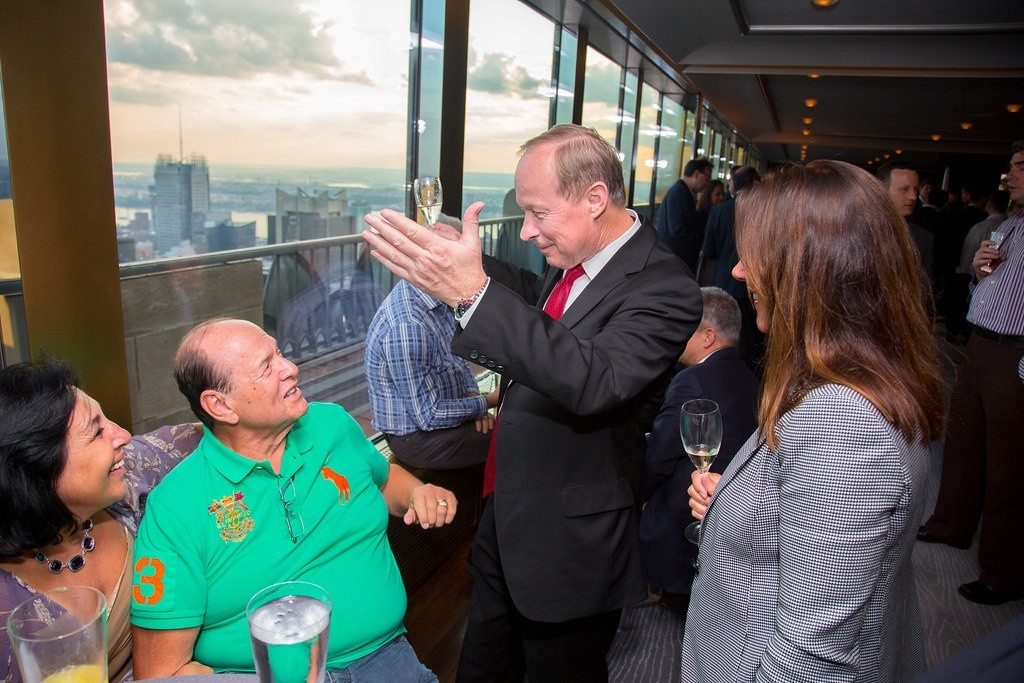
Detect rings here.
[437,498,448,508]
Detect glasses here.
[702,171,712,181]
[277,473,305,543]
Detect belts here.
[974,324,1024,349]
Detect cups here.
[414,176,442,233]
[247,580,332,683]
[5,587,111,683]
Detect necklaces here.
[33,517,95,574]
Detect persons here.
[659,156,1008,348]
[645,287,764,606]
[130,316,456,683]
[680,160,951,683]
[915,140,1024,605]
[364,279,500,470]
[0,358,136,683]
[361,124,703,683]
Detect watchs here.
[454,277,489,318]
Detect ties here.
[482,262,584,499]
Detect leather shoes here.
[958,581,1024,605]
[916,525,974,550]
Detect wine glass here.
[679,398,720,544]
[980,232,1005,272]
